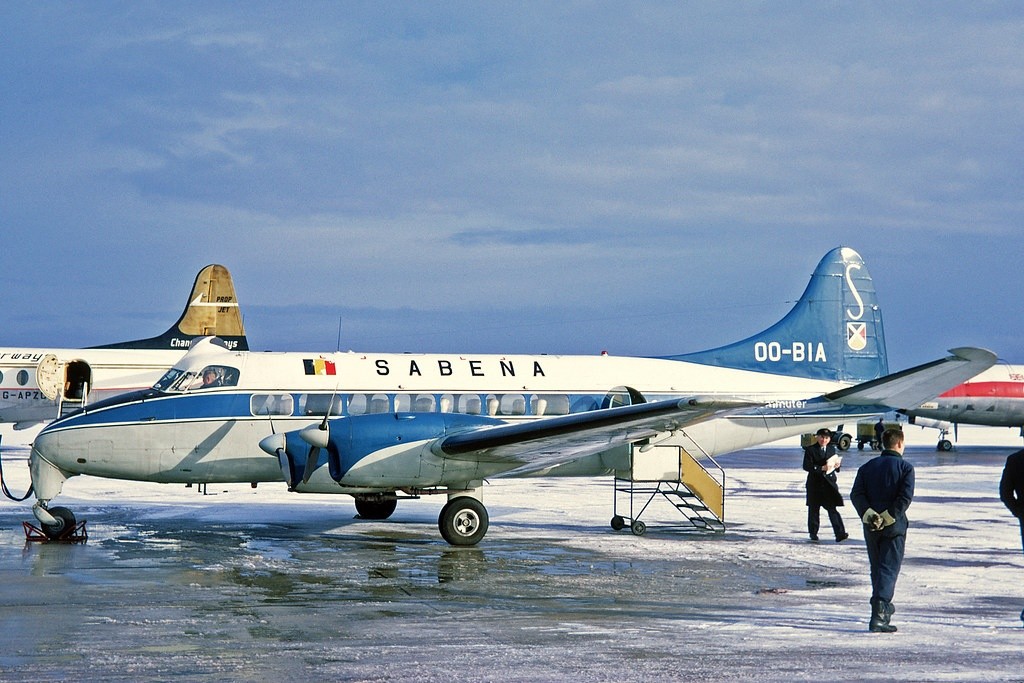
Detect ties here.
[822,447,825,458]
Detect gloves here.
[861,508,884,531]
[868,510,896,531]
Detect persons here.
[200,370,219,388]
[999,447,1024,628]
[850,429,915,633]
[803,428,849,542]
[874,418,885,451]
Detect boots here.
[869,601,897,633]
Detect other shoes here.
[836,533,849,542]
[810,534,819,540]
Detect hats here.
[817,428,833,437]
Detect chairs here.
[270,399,292,415]
[466,399,481,414]
[531,398,547,415]
[440,398,450,413]
[327,398,343,415]
[511,399,525,415]
[381,399,400,413]
[486,398,499,416]
[370,399,386,413]
[416,398,432,411]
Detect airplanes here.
[894,362,1024,453]
[0,246,1002,551]
